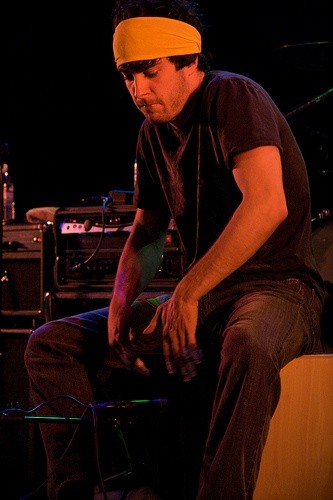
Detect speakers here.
[0,222,59,500]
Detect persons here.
[24,0,325,500]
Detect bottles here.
[2,164,14,220]
